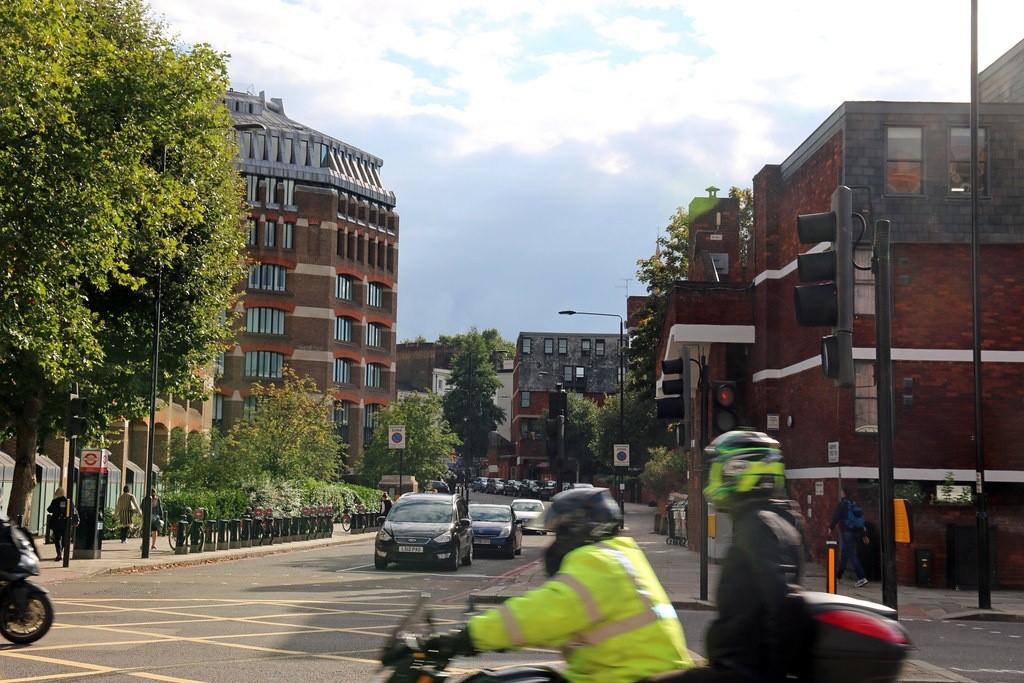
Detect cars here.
[537,480,558,501]
[471,477,488,493]
[503,479,521,496]
[519,479,538,498]
[511,499,548,536]
[467,504,523,560]
[486,478,505,495]
[560,482,593,492]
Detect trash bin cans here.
[671,500,688,547]
[708,503,734,565]
[665,501,675,545]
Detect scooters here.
[0,507,54,645]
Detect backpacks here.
[840,498,865,532]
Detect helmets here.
[538,486,627,575]
[701,430,788,508]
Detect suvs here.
[374,492,475,571]
[422,455,465,494]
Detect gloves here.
[381,632,434,670]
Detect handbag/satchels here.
[0,518,42,582]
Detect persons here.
[448,475,457,494]
[114,485,142,543]
[636,426,808,683]
[828,486,869,587]
[379,492,394,527]
[46,486,80,562]
[424,487,698,683]
[185,507,193,546]
[436,479,450,493]
[140,489,164,550]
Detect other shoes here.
[55,555,62,562]
[151,546,157,550]
[855,578,869,588]
[836,578,841,585]
[139,545,143,550]
[121,540,128,544]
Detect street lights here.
[538,371,565,482]
[466,348,510,500]
[140,256,163,559]
[559,311,625,529]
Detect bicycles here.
[340,499,367,533]
[169,513,205,551]
[241,510,334,545]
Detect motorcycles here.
[371,583,912,683]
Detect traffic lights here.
[710,382,741,436]
[674,423,691,452]
[793,210,839,328]
[821,336,842,377]
[653,346,691,421]
[65,393,88,438]
